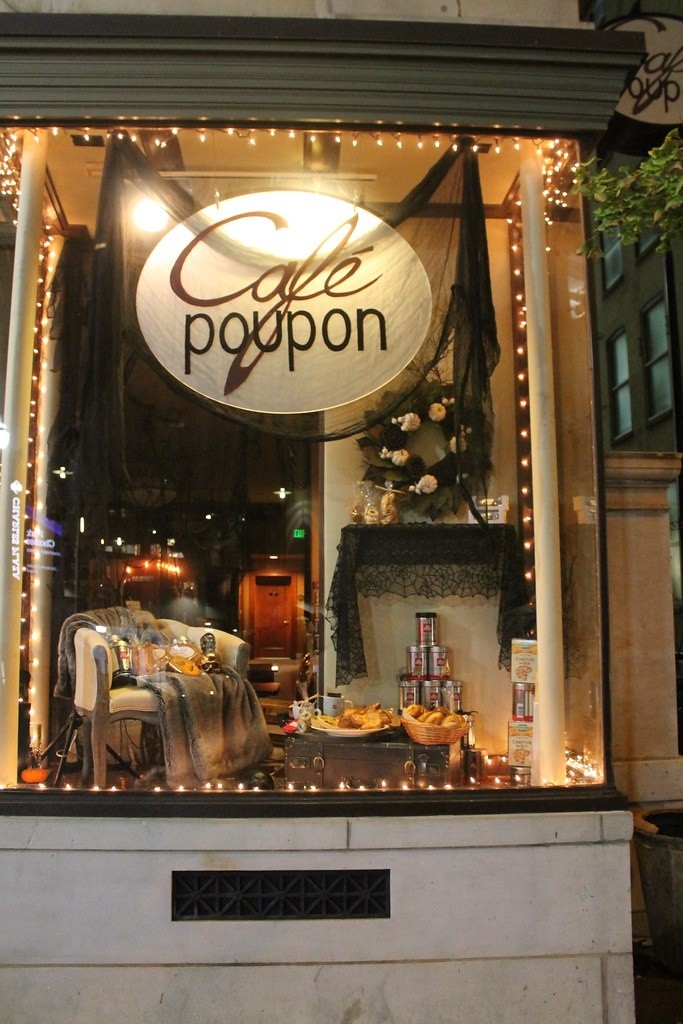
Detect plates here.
[311,724,390,737]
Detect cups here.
[463,715,476,747]
[291,703,321,720]
[463,748,487,787]
[398,680,462,714]
[405,647,452,680]
[508,767,532,785]
[414,611,438,646]
[512,682,534,722]
[321,694,353,716]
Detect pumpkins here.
[21,764,48,783]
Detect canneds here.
[398,612,462,716]
[108,640,132,675]
[512,683,535,722]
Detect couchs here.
[72,619,251,791]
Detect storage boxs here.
[507,718,534,767]
[284,727,463,788]
[511,638,538,683]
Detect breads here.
[338,702,392,730]
[405,704,465,727]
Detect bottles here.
[104,635,133,686]
[307,666,318,697]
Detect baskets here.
[401,708,468,746]
